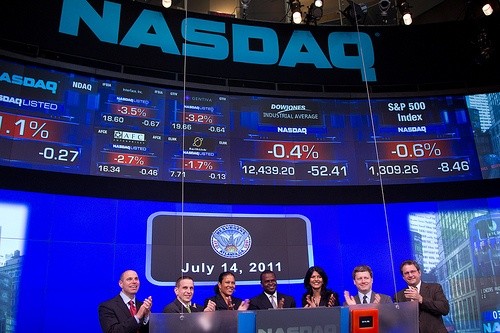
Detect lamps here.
[161,0,494,26]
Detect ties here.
[270,295,276,308]
[127,300,137,315]
[186,306,193,313]
[362,296,368,304]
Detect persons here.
[344,265,399,333]
[247,270,296,311]
[394,259,450,333]
[204,272,250,311]
[302,266,340,308]
[162,276,217,313]
[98,269,152,333]
